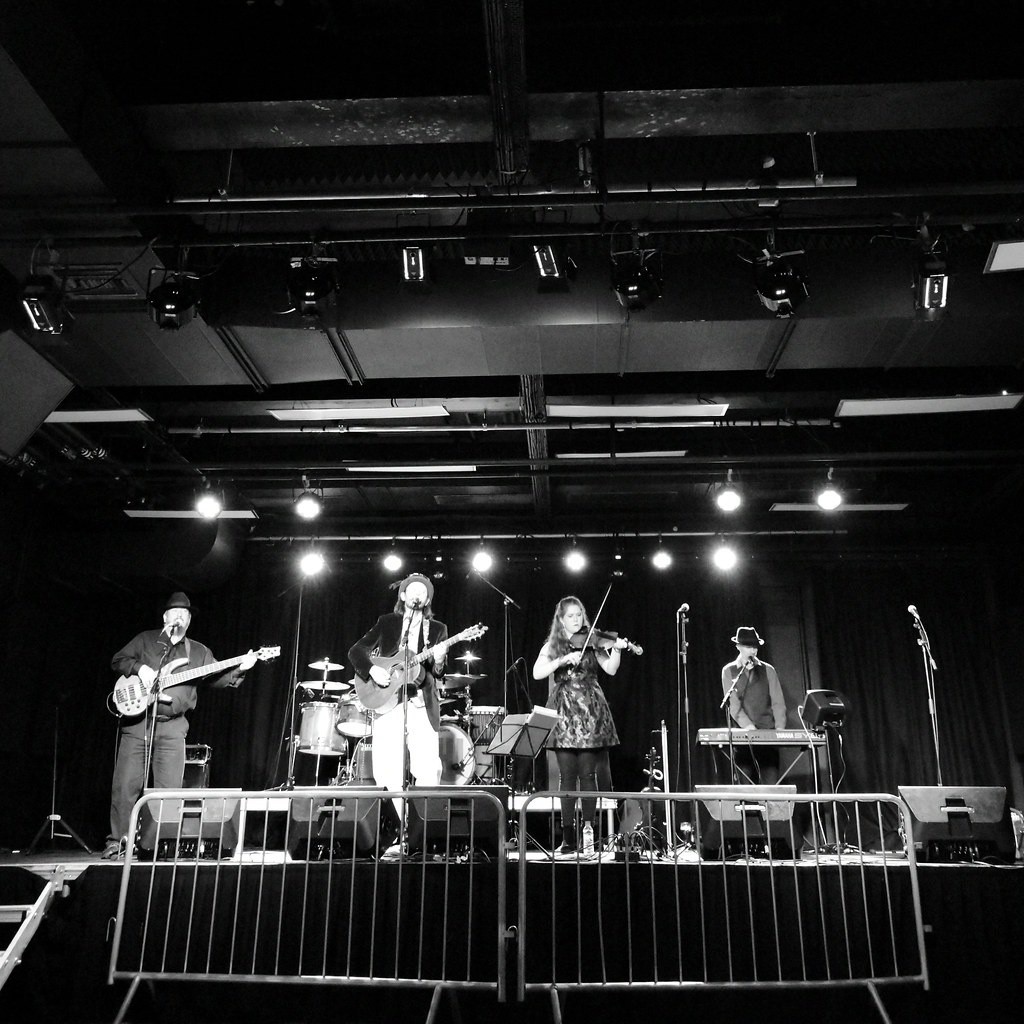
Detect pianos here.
[695,727,835,851]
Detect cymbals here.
[453,655,482,660]
[436,674,487,689]
[300,680,351,691]
[307,662,345,671]
[348,679,355,685]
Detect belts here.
[146,713,181,722]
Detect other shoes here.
[103,840,125,859]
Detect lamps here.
[911,224,949,326]
[145,247,204,330]
[757,228,812,321]
[610,233,659,314]
[981,218,1024,274]
[290,247,341,323]
[24,270,78,337]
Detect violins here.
[639,746,666,850]
[568,625,644,656]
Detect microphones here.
[678,603,689,612]
[320,554,332,573]
[171,619,183,627]
[748,656,755,666]
[507,657,521,674]
[413,596,421,604]
[466,567,474,578]
[907,605,919,619]
[451,762,465,770]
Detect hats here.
[731,628,765,646]
[164,592,191,609]
[399,574,433,606]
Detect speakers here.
[137,788,242,859]
[404,785,510,862]
[287,786,401,860]
[897,786,1015,866]
[693,785,804,861]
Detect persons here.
[101,590,258,859]
[723,628,786,783]
[348,573,448,859]
[532,597,628,852]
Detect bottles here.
[582,821,595,858]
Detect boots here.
[562,824,576,853]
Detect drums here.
[297,694,371,756]
[468,705,508,744]
[438,722,476,786]
[438,694,472,726]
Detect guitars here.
[112,643,281,717]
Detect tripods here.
[23,708,91,854]
[482,723,555,861]
